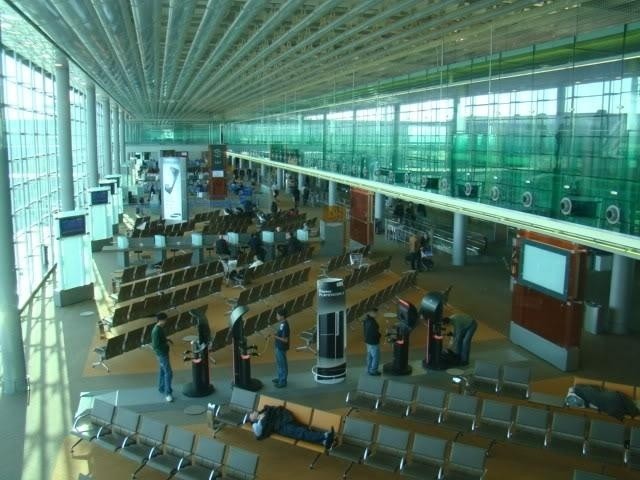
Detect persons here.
[249,406,335,449]
[391,201,430,269]
[569,384,640,421]
[442,314,478,365]
[214,168,311,288]
[151,313,175,401]
[365,307,382,376]
[272,308,290,388]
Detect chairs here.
[129,154,279,202]
[68,395,260,480]
[90,206,454,374]
[344,357,640,464]
[211,386,491,479]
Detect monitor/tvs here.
[100,177,120,194]
[59,214,85,239]
[91,190,108,205]
